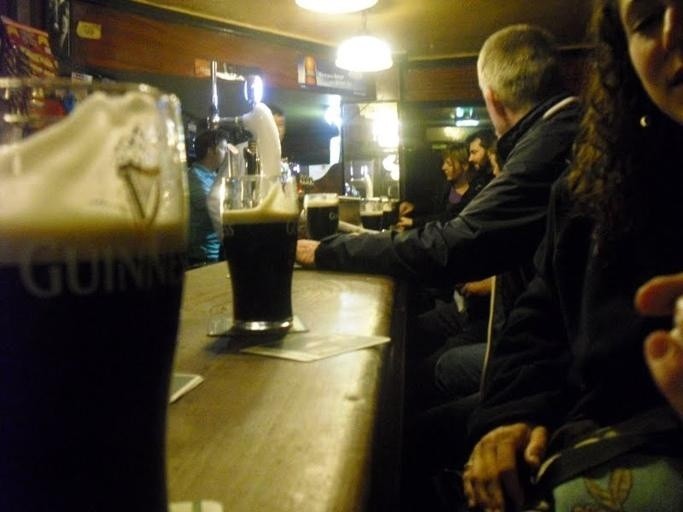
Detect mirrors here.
[337,98,403,214]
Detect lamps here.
[333,10,394,75]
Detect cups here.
[1,78,189,512]
[358,198,398,231]
[303,192,338,243]
[221,173,299,333]
